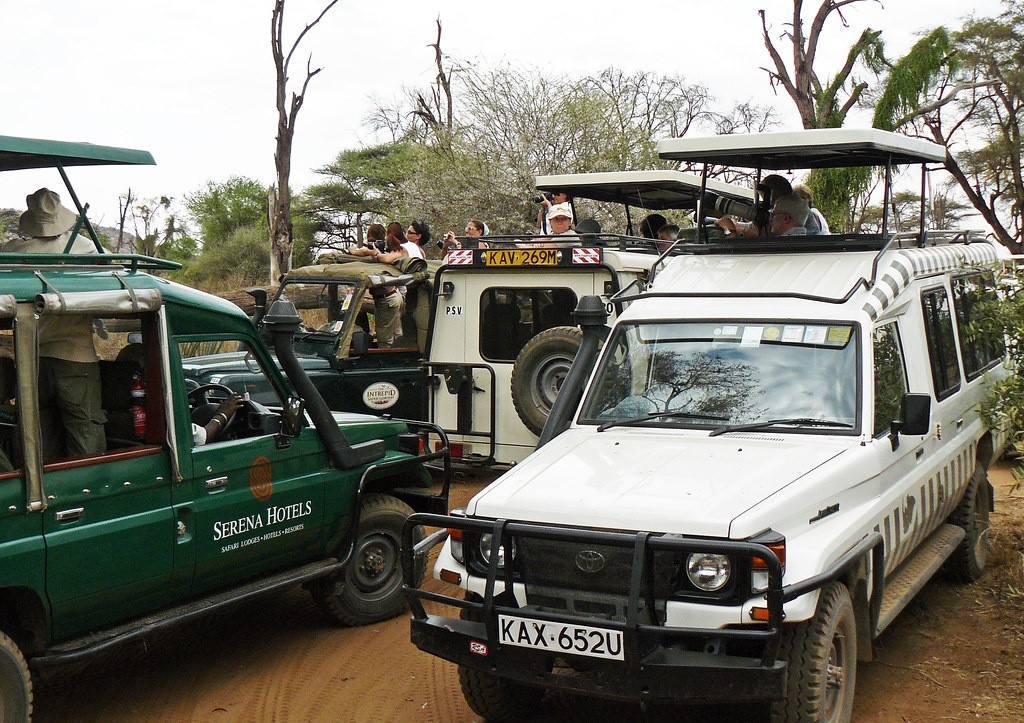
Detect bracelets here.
[215,412,229,424]
[210,418,222,433]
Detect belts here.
[374,286,398,298]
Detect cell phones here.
[446,233,448,239]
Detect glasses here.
[408,230,417,234]
[771,211,783,217]
[466,226,476,229]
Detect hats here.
[482,222,490,236]
[20,189,76,237]
[548,204,572,219]
[412,220,430,245]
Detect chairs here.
[98,360,145,443]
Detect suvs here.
[238,252,439,451]
[418,173,832,476]
[401,126,1019,722]
[0,136,456,723]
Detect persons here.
[114,342,245,447]
[0,186,115,467]
[347,172,830,358]
[1,356,18,415]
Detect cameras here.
[436,240,450,251]
[368,240,385,253]
[700,184,772,224]
[532,192,552,203]
